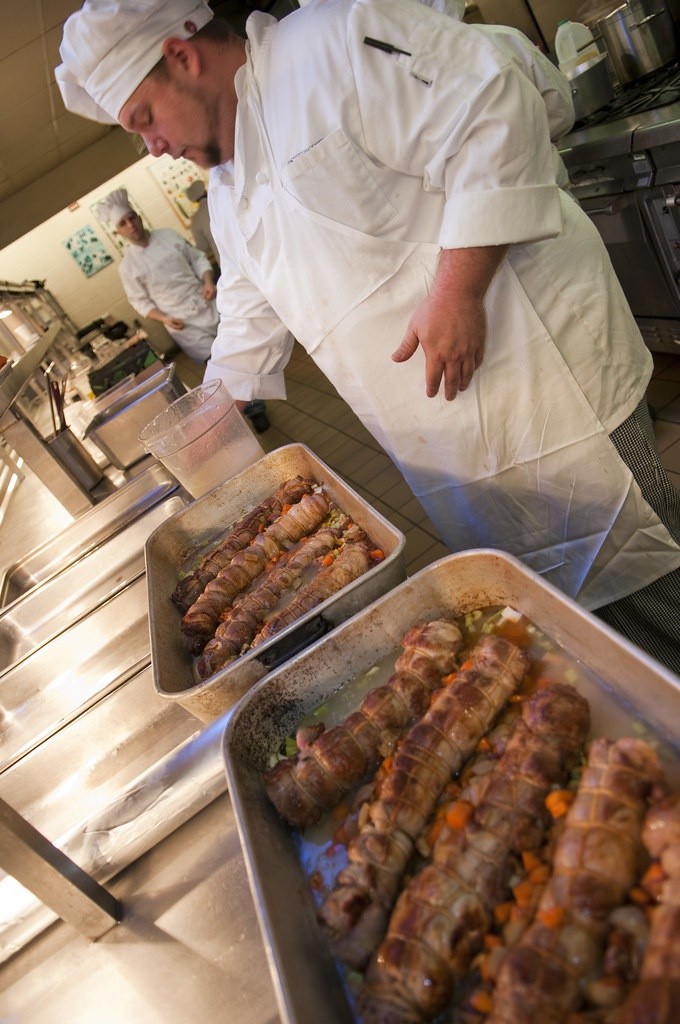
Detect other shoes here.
[250,411,271,434]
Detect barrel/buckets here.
[44,426,105,491]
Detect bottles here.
[553,18,599,70]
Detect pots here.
[597,1,679,84]
[564,51,613,121]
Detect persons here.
[420,1,579,208]
[98,187,271,435]
[56,1,680,679]
[187,180,220,271]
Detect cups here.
[138,377,266,500]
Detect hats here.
[91,188,133,231]
[57,0,216,124]
[188,181,205,201]
